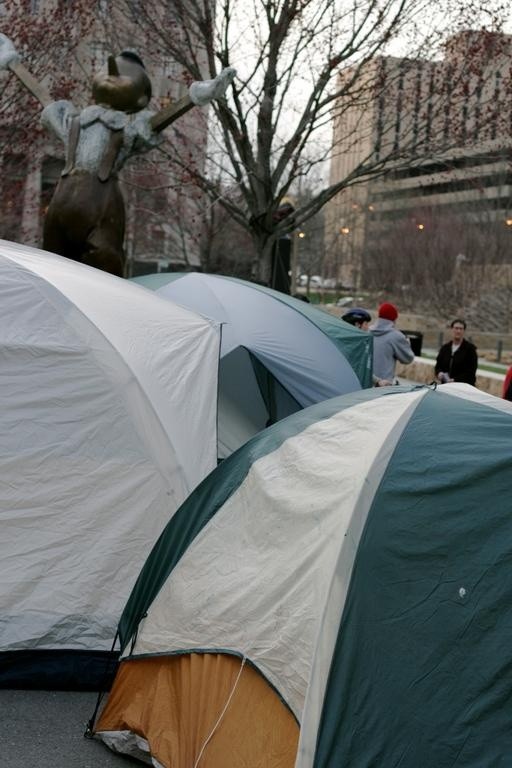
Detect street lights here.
[291,230,307,294]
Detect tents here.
[126,269,383,467]
[1,233,224,695]
[80,383,512,768]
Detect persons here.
[0,28,237,275]
[367,299,415,385]
[337,306,392,389]
[433,318,479,388]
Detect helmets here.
[341,308,372,323]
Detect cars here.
[323,296,363,307]
[288,271,359,293]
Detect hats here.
[377,303,398,321]
